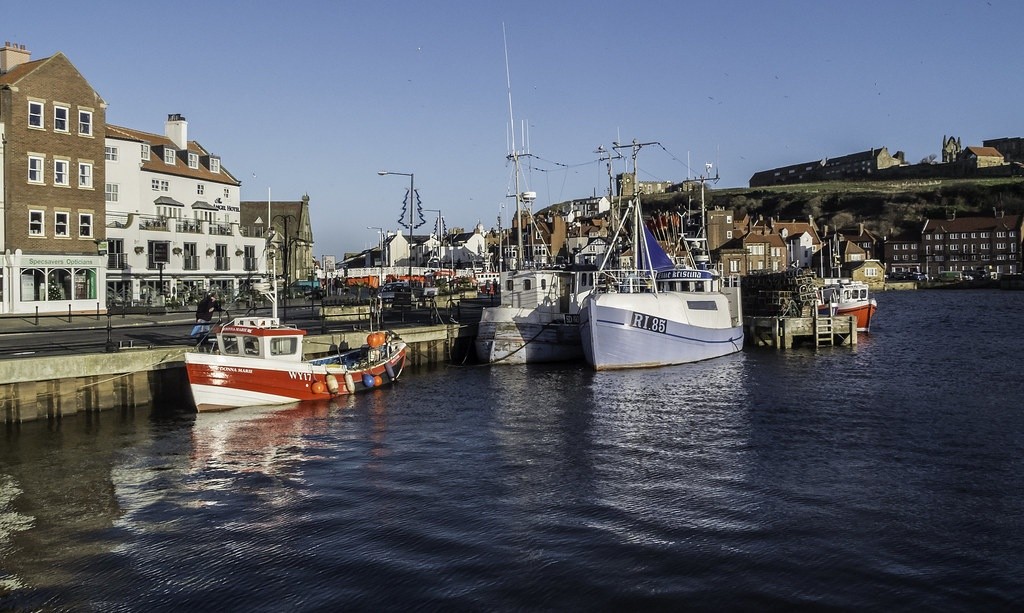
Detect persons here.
[191,292,222,345]
[334,274,343,295]
[830,289,840,316]
[486,278,498,298]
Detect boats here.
[185,313,407,412]
[818,233,877,336]
[473,20,745,374]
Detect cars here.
[285,279,322,299]
[378,281,416,303]
[478,284,497,296]
[891,269,992,283]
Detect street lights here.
[269,213,300,324]
[423,209,442,267]
[366,226,384,288]
[377,170,415,293]
